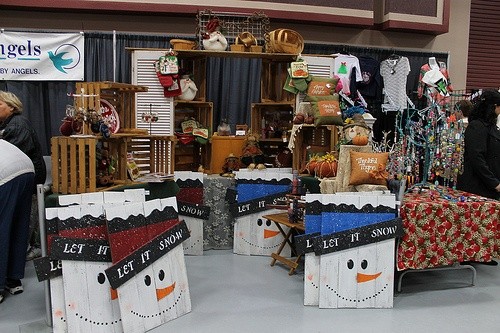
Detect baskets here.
[270,28,304,54]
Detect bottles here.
[312,127,327,146]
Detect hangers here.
[340,45,351,55]
[387,49,400,59]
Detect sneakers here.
[0,279,24,303]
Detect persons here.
[0,138,35,303]
[0,90,47,261]
[436,90,500,264]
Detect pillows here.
[349,149,388,185]
[303,76,342,101]
[309,94,343,127]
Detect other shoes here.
[26,244,42,260]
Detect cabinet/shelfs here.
[52,47,338,193]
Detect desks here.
[263,211,306,274]
[396,187,500,292]
